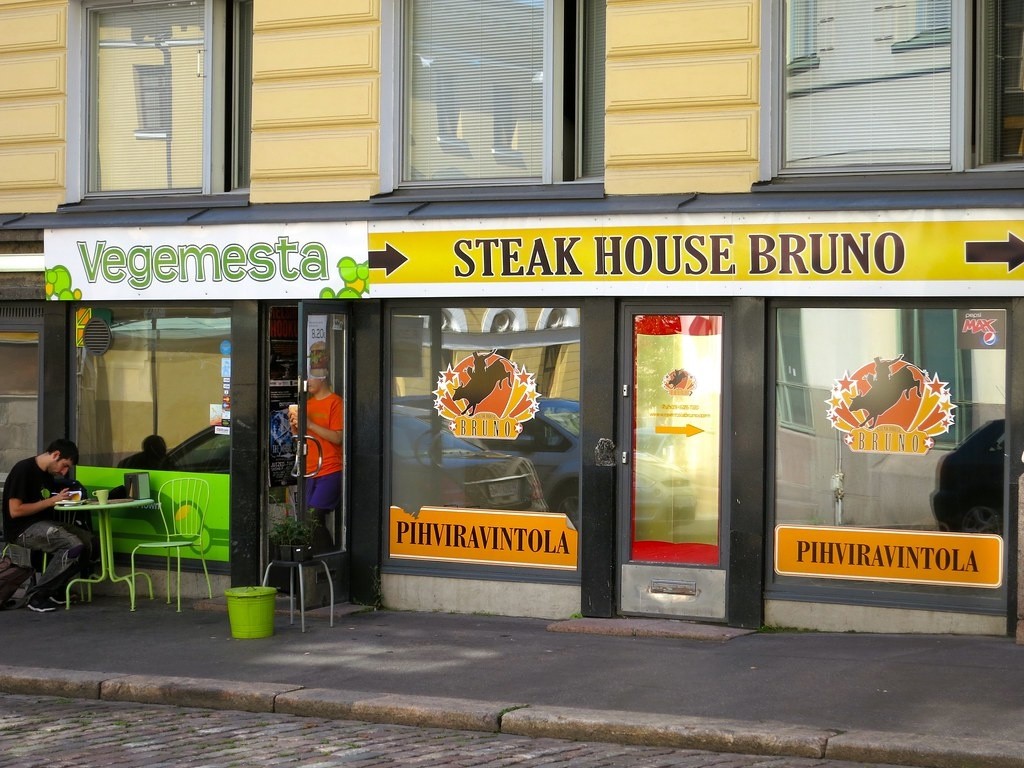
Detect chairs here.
[131,478,213,613]
[3,508,85,604]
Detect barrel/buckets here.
[223,587,277,639]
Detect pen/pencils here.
[51,492,59,494]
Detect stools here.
[262,559,334,634]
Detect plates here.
[55,502,80,505]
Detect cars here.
[168,394,697,541]
[929,419,1004,533]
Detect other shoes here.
[26,599,56,612]
[49,596,71,605]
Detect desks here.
[54,499,155,612]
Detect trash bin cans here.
[225,586,278,639]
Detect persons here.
[117,434,177,471]
[287,366,346,553]
[2,438,84,613]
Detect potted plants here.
[265,493,320,562]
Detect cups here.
[289,404,299,422]
[92,490,109,505]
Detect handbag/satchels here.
[0,542,31,604]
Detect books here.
[63,491,82,501]
